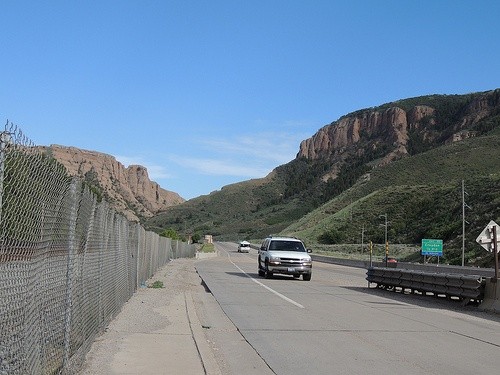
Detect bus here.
[239,241,250,253]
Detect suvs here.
[257,237,312,281]
